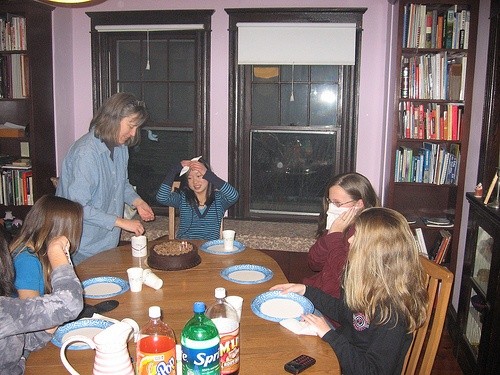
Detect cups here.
[131,236,147,257]
[127,267,144,292]
[142,269,163,291]
[222,230,235,251]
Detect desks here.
[24,240,342,375]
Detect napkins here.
[277,319,318,337]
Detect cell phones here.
[284,355,315,375]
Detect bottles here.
[205,287,240,375]
[136,305,177,375]
[181,301,220,374]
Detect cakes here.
[147,239,200,271]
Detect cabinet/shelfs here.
[0,0,57,213]
[454,192,500,375]
[384,0,479,284]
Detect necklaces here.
[198,200,205,206]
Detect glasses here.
[327,197,353,207]
[129,100,146,108]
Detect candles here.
[179,241,188,253]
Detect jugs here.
[60,323,135,375]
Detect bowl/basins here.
[472,296,487,312]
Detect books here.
[407,217,455,264]
[0,13,31,99]
[0,159,34,206]
[393,4,471,185]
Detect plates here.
[250,292,315,323]
[225,295,244,325]
[50,319,113,350]
[221,265,273,284]
[82,276,129,299]
[200,240,246,255]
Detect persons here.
[156,158,239,240]
[269,207,429,375]
[55,93,154,268]
[301,173,380,329]
[0,194,84,375]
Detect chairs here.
[401,256,453,375]
[168,181,223,240]
